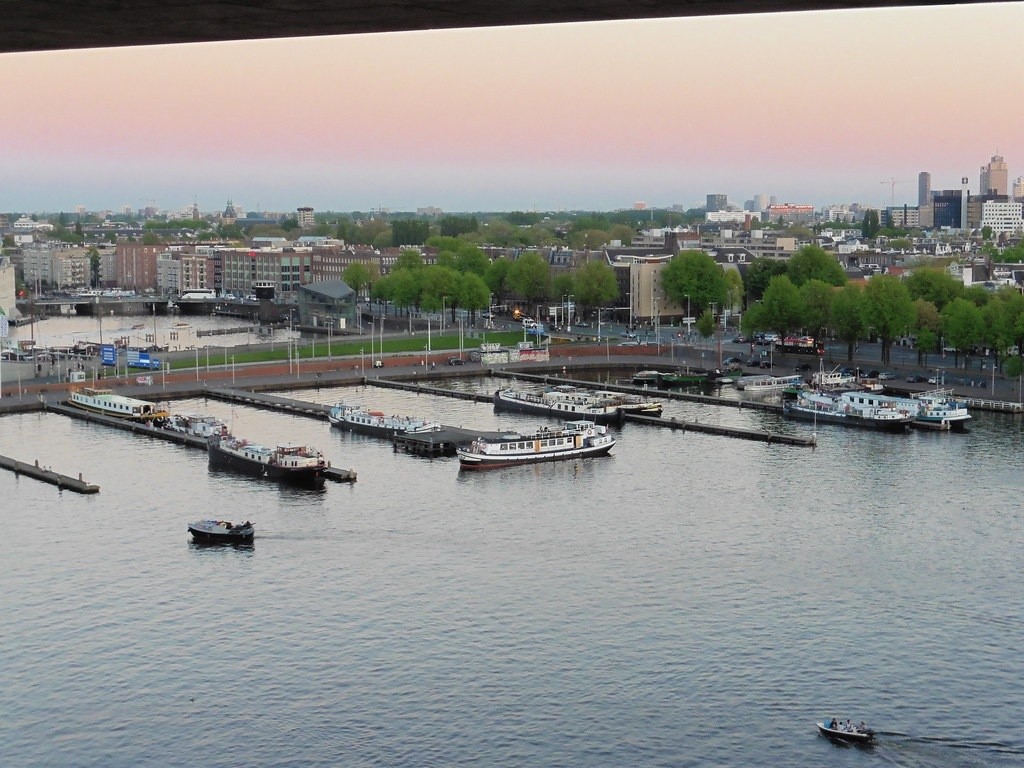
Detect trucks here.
[770,335,827,356]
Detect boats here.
[455,409,617,467]
[66,383,327,482]
[815,722,874,743]
[186,518,255,543]
[327,399,442,438]
[495,353,972,427]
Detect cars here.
[511,310,562,333]
[222,292,260,302]
[928,375,942,385]
[746,358,761,367]
[482,312,496,319]
[878,372,897,381]
[758,360,777,369]
[733,333,777,346]
[859,370,879,379]
[449,358,466,366]
[905,375,927,384]
[941,345,957,352]
[722,356,741,365]
[793,363,811,373]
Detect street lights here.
[367,315,375,368]
[684,294,691,339]
[488,292,495,327]
[567,295,575,327]
[287,309,297,359]
[940,314,950,360]
[356,306,362,335]
[326,316,334,338]
[442,296,450,330]
[294,324,302,365]
[561,294,568,328]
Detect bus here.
[179,289,217,301]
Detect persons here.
[859,721,867,731]
[842,719,853,732]
[830,718,837,730]
[837,722,844,731]
